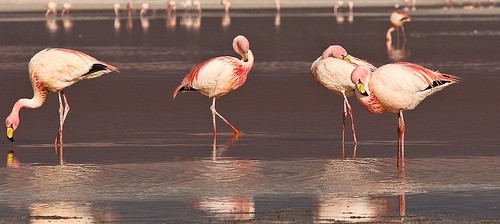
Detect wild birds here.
[114,0,232,16]
[44,2,71,15]
[4,46,120,145]
[385,11,411,43]
[333,0,354,14]
[310,44,378,145]
[172,34,254,146]
[275,0,281,12]
[350,60,461,170]
